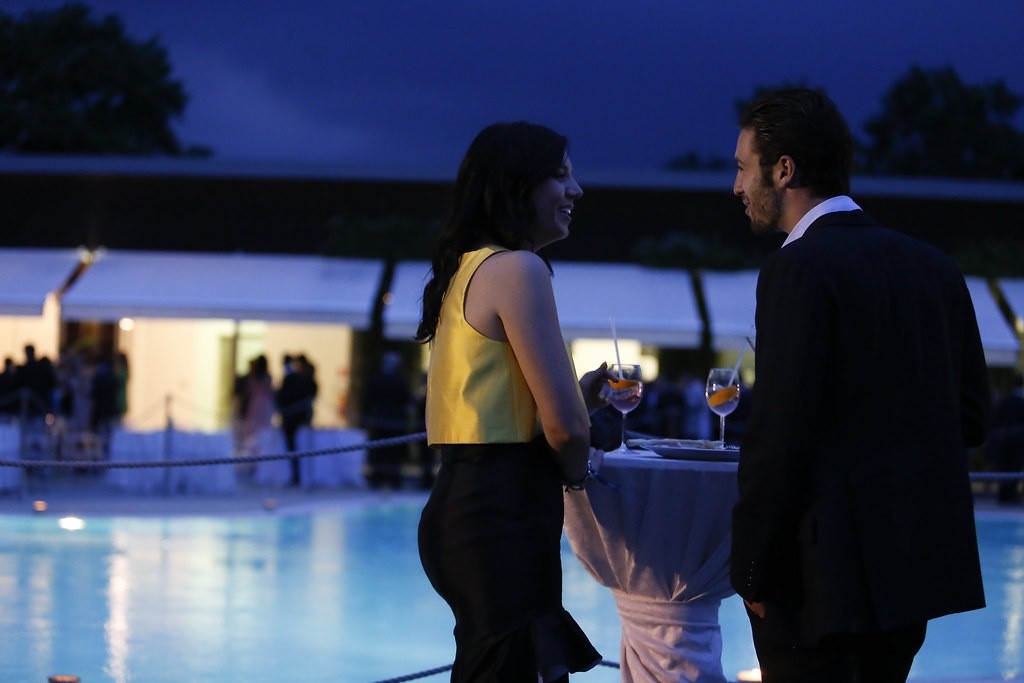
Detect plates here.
[626,438,740,461]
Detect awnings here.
[0,247,1024,365]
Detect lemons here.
[607,379,637,388]
[709,385,736,406]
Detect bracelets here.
[565,458,592,494]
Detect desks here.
[562,448,740,683]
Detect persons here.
[0,343,129,477]
[983,375,1024,505]
[414,122,620,683]
[364,350,751,492]
[233,351,317,486]
[729,88,994,683]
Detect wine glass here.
[604,364,643,457]
[705,368,740,449]
